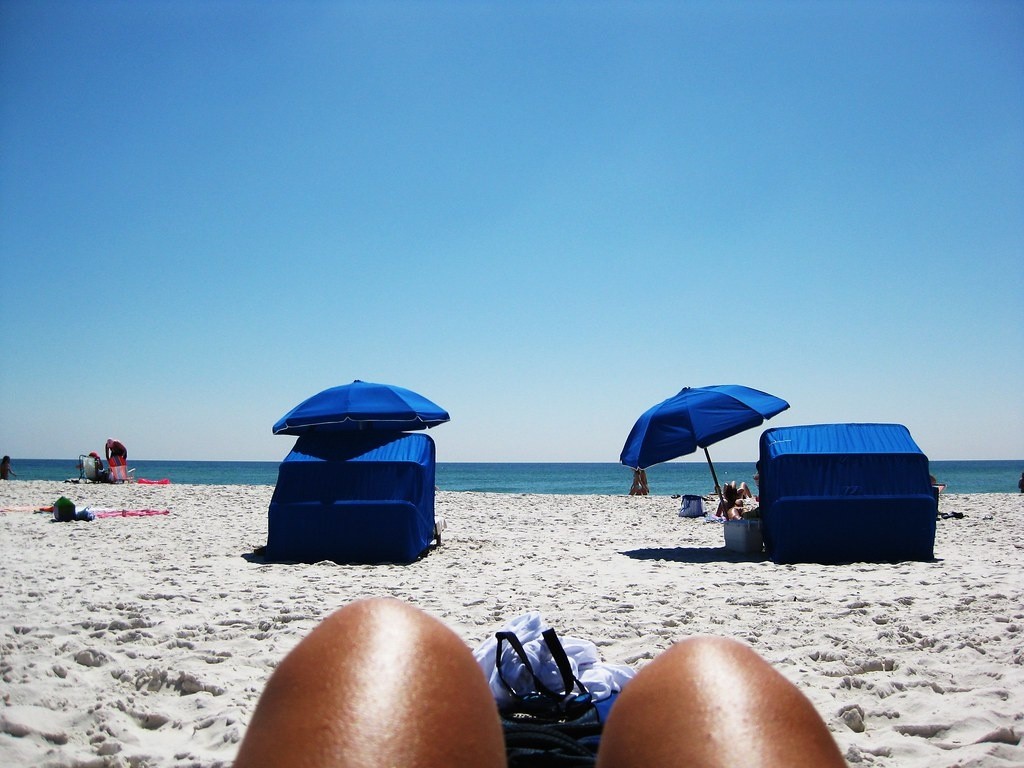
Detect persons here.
[77,453,105,476]
[754,461,760,487]
[0,456,16,480]
[716,481,751,519]
[629,468,648,495]
[234,597,848,768]
[106,439,127,482]
[1019,473,1024,493]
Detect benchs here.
[263,431,448,565]
[745,424,940,562]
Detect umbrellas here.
[620,384,790,521]
[272,380,450,435]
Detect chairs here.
[932,484,947,492]
[107,465,136,483]
[78,455,108,484]
